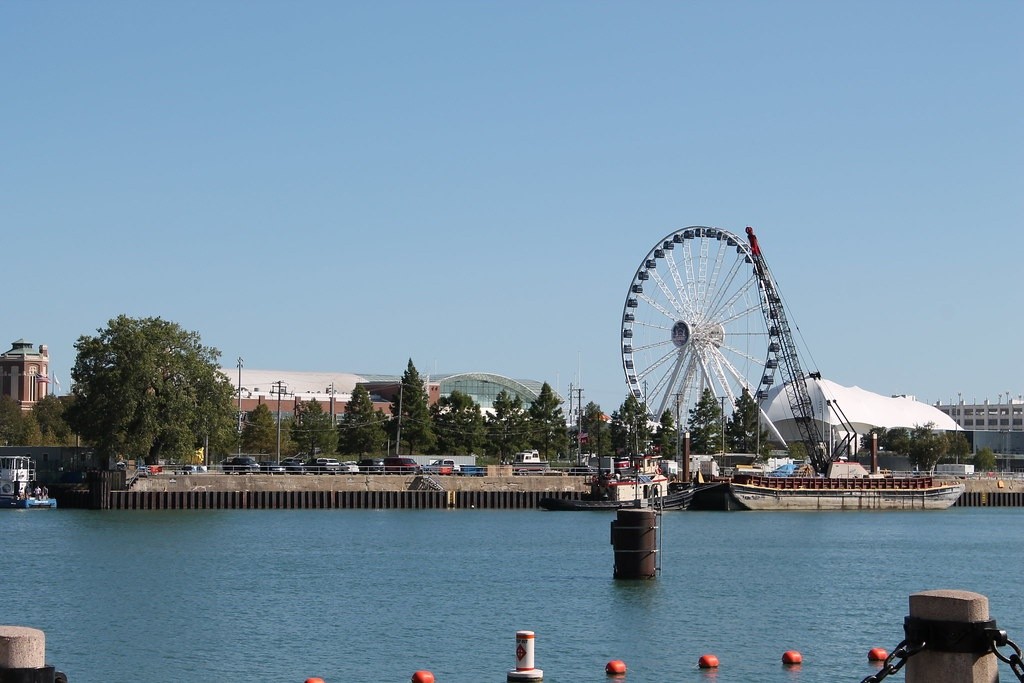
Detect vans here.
[431,459,455,474]
[383,456,422,475]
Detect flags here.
[34,371,61,391]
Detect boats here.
[542,473,697,512]
[0,456,59,510]
[728,433,966,510]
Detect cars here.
[258,462,286,476]
[462,465,485,476]
[340,461,360,476]
[279,456,339,475]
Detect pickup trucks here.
[221,457,261,474]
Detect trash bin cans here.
[476,468,484,476]
[462,464,476,476]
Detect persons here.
[654,486,658,497]
[18,485,48,501]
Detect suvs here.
[357,458,387,476]
